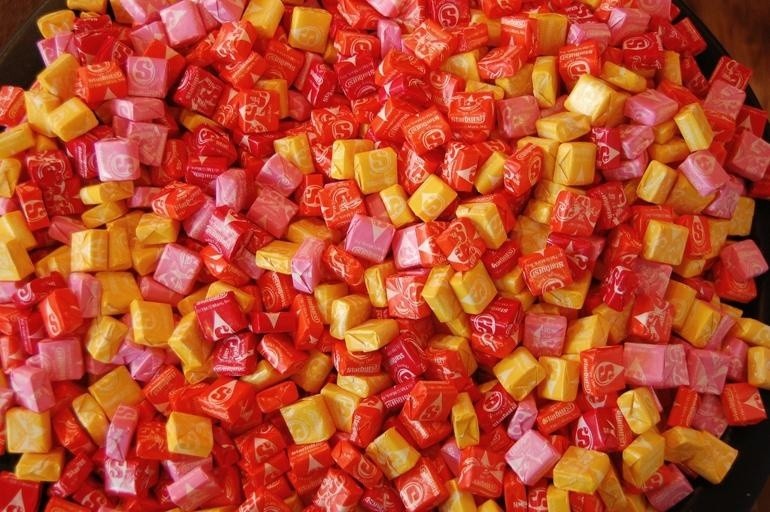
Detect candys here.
[0,1,770,512]
